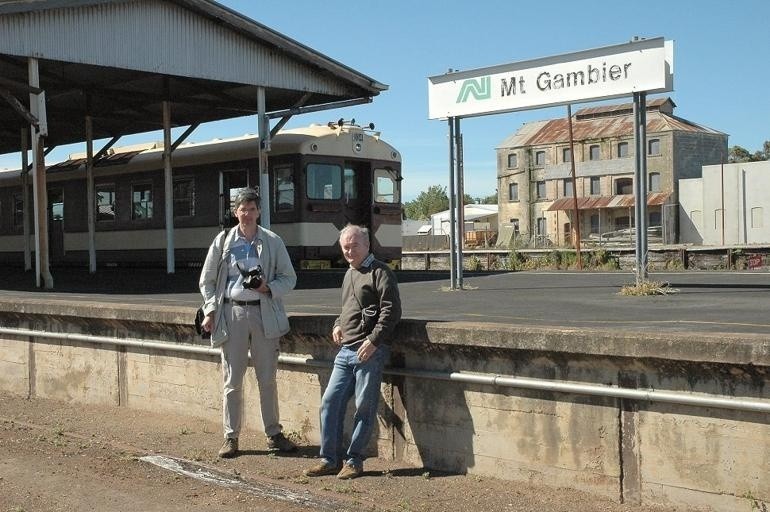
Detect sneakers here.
[267,433,296,452]
[218,437,238,458]
[338,462,363,480]
[306,461,338,477]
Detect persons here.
[304,222,402,479]
[199,187,297,457]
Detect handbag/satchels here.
[362,306,398,339]
[194,304,213,339]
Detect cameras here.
[232,261,263,289]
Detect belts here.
[224,296,261,307]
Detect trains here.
[0,119,401,273]
